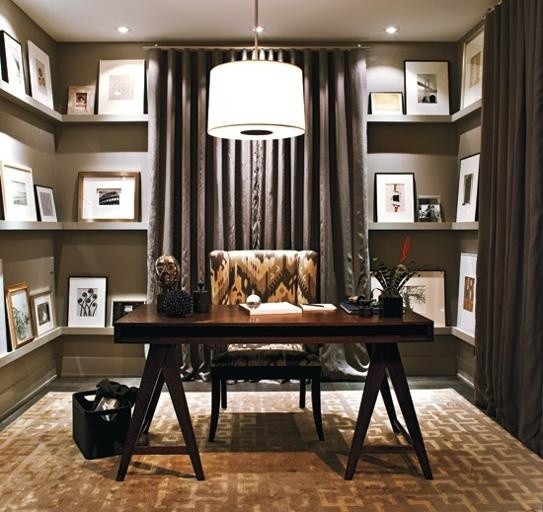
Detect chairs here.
[206,249,326,444]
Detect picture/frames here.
[67,84,95,119]
[405,61,450,116]
[29,286,58,338]
[417,194,442,222]
[1,162,39,221]
[110,295,147,327]
[375,173,415,224]
[456,251,478,338]
[35,187,58,222]
[4,283,36,350]
[97,57,146,117]
[77,170,142,221]
[371,267,448,328]
[455,152,480,222]
[0,29,29,96]
[368,91,404,116]
[26,38,57,111]
[460,25,485,110]
[67,276,106,326]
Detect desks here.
[113,303,435,483]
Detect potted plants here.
[366,257,427,319]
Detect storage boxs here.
[71,389,132,460]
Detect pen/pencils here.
[303,304,324,307]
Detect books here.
[339,300,380,315]
[238,301,303,316]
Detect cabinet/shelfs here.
[367,101,484,345]
[0,77,156,420]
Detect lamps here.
[208,0,304,143]
